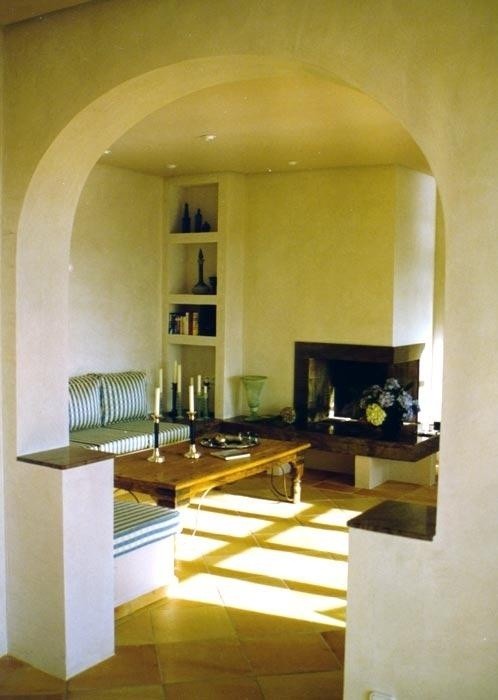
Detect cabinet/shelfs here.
[159,170,248,421]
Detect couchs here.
[68,371,191,458]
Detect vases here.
[368,422,394,440]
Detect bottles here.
[181,200,212,233]
[191,247,211,295]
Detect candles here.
[152,358,208,418]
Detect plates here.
[195,433,263,450]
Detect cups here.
[207,275,216,295]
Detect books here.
[211,449,250,461]
[170,310,201,336]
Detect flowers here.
[357,377,421,432]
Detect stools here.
[114,501,182,621]
